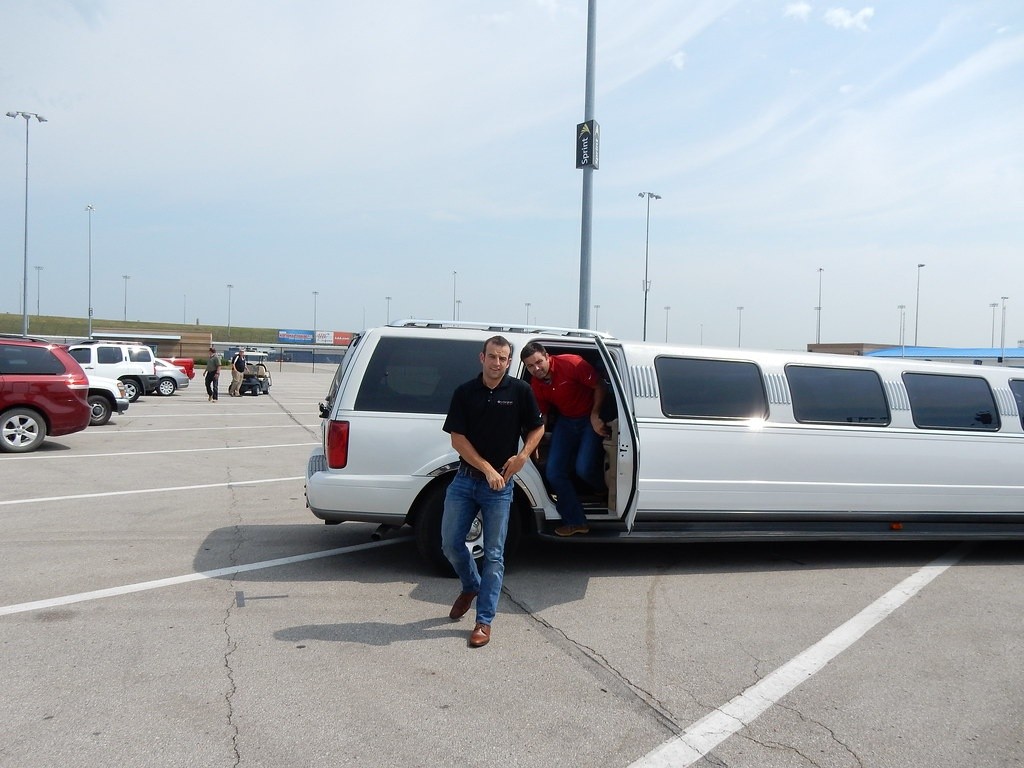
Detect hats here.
[209,348,216,352]
[239,347,245,352]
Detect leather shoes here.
[471,623,490,646]
[449,591,477,619]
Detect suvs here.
[65,340,161,403]
[0,333,92,453]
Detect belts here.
[460,465,486,480]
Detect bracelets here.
[216,373,219,375]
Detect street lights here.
[593,304,601,331]
[638,191,662,341]
[226,282,233,327]
[736,305,745,348]
[6,111,49,337]
[914,263,926,346]
[312,289,320,329]
[897,304,906,346]
[524,303,532,325]
[664,307,672,343]
[83,204,95,341]
[451,269,462,321]
[34,266,44,316]
[814,267,825,344]
[384,295,393,325]
[121,274,132,321]
[988,296,1009,348]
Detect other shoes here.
[554,521,589,535]
[237,395,242,397]
[208,395,213,401]
[230,393,233,397]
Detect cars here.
[154,358,190,396]
[164,358,197,380]
[304,318,1024,579]
[86,375,129,426]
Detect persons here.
[203,348,221,401]
[440,335,544,647]
[521,343,609,535]
[229,348,249,397]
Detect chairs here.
[245,363,269,381]
